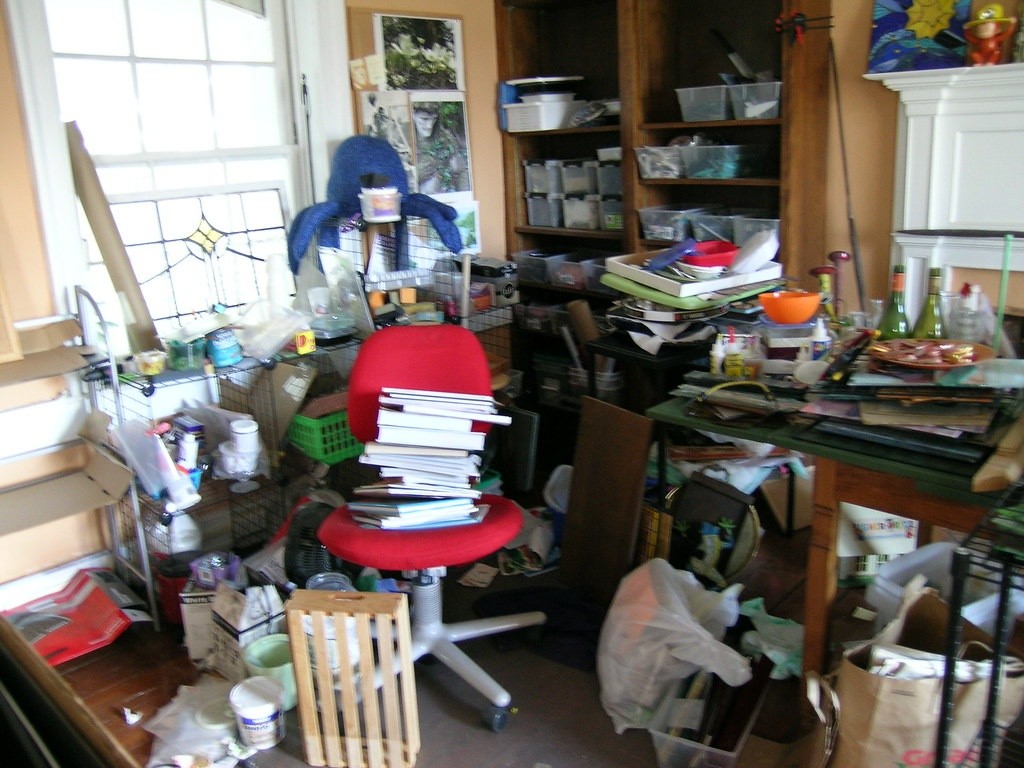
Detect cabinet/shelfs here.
[0,282,162,638]
[493,0,833,316]
[93,304,518,644]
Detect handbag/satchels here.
[824,573,1024,768]
[670,379,802,420]
[739,597,805,679]
[595,558,752,735]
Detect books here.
[348,387,512,528]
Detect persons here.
[414,102,468,194]
[963,3,1017,67]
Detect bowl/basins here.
[164,339,208,370]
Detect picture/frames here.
[407,88,475,205]
[372,11,466,94]
[360,92,419,194]
[429,201,483,256]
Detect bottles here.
[878,264,911,343]
[912,263,952,342]
[154,502,204,623]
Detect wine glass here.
[218,439,262,493]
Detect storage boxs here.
[502,100,587,133]
[209,581,285,687]
[283,408,365,467]
[732,209,780,248]
[523,165,561,194]
[579,257,610,294]
[687,207,753,243]
[526,198,564,227]
[518,93,577,103]
[636,203,723,243]
[596,147,623,163]
[282,589,422,768]
[725,81,783,121]
[598,200,624,232]
[544,249,611,290]
[171,415,208,449]
[188,550,241,590]
[561,198,600,230]
[861,535,1024,647]
[510,245,589,285]
[688,470,782,538]
[675,85,733,122]
[470,281,492,310]
[759,328,810,362]
[678,143,770,180]
[512,296,575,335]
[436,254,520,308]
[560,166,597,195]
[177,563,248,667]
[759,466,816,535]
[633,145,686,180]
[648,605,779,768]
[836,500,920,590]
[596,167,624,196]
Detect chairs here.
[315,324,562,739]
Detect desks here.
[646,395,1024,735]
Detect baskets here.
[286,407,366,467]
[567,362,625,391]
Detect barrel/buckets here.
[241,633,299,713]
[229,675,287,752]
